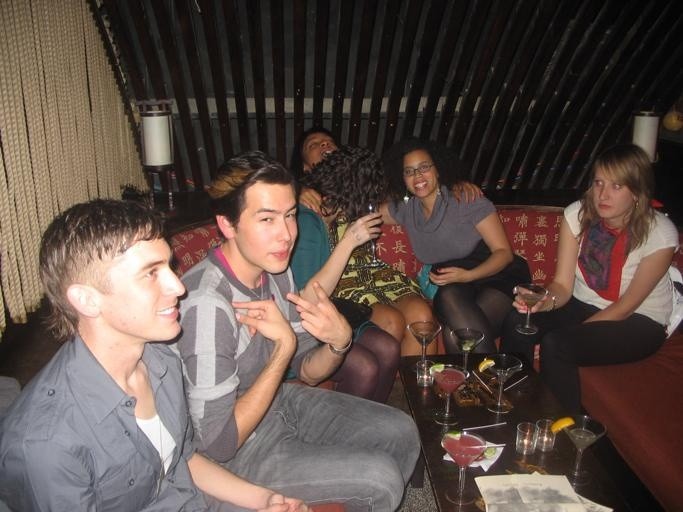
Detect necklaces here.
[240,274,264,300]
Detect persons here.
[512,142,680,408]
[165,149,421,512]
[289,143,401,405]
[374,126,532,365]
[0,199,312,512]
[297,127,485,357]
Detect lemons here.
[441,430,462,448]
[478,359,495,372]
[552,417,576,434]
[429,362,445,375]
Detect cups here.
[514,421,539,456]
[414,359,436,389]
[535,419,556,453]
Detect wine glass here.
[483,353,523,414]
[515,284,548,336]
[407,320,442,361]
[427,362,470,427]
[449,327,485,374]
[359,199,386,269]
[439,432,487,506]
[560,411,608,488]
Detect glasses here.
[402,163,435,177]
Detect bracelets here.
[327,339,352,356]
[548,295,557,312]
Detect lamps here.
[632,110,661,167]
[137,100,175,210]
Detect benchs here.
[532,327,683,510]
[169,201,569,354]
